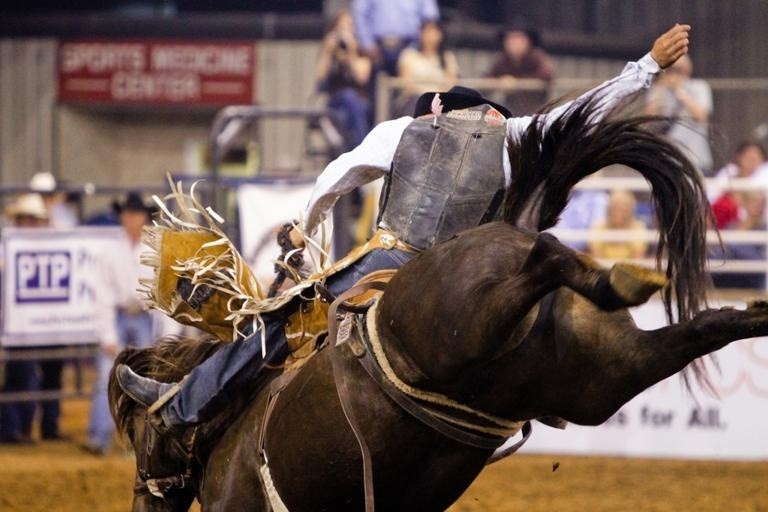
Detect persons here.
[77,188,182,456]
[552,166,611,254]
[304,0,555,157]
[640,53,715,177]
[587,188,649,261]
[113,22,694,427]
[0,192,73,444]
[29,171,80,228]
[705,139,768,289]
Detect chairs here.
[5,431,102,454]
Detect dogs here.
[106,75,768,511]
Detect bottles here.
[413,85,515,119]
[5,172,57,221]
[113,194,158,214]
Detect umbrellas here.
[115,362,163,414]
